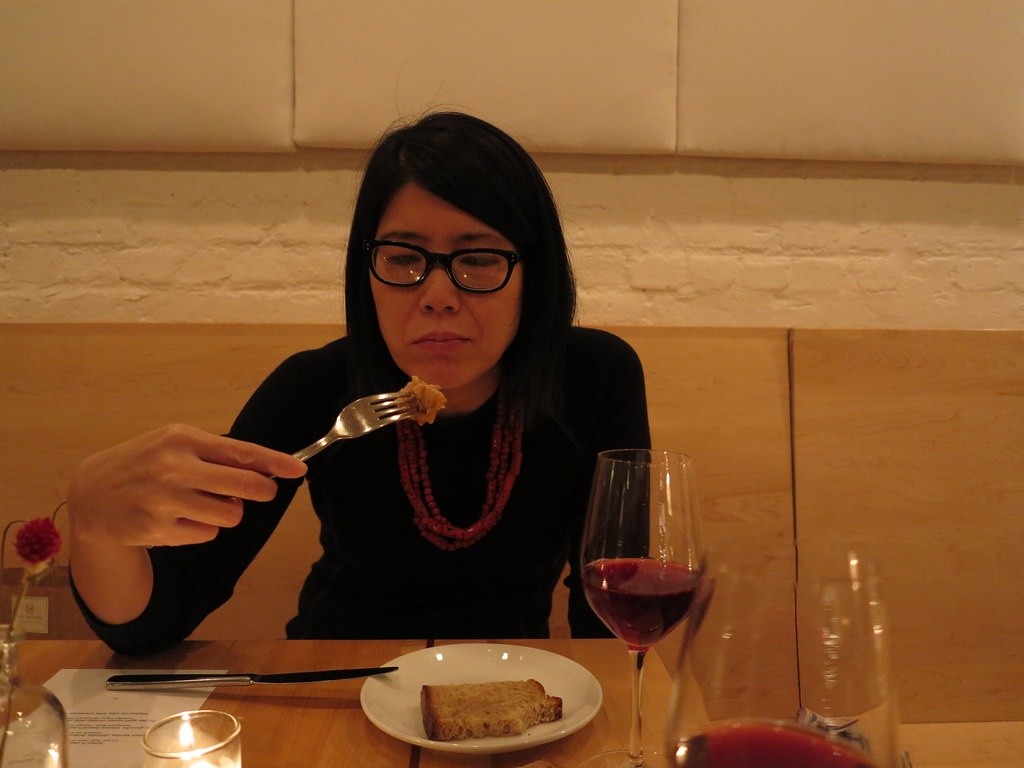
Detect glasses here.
[365,240,525,293]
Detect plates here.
[358,641,606,756]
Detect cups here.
[664,525,907,768]
[143,709,245,768]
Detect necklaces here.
[397,402,522,550]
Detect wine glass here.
[581,446,716,768]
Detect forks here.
[254,392,424,480]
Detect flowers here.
[5,515,62,641]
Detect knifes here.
[102,664,397,690]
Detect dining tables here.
[1,638,711,768]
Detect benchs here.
[3,320,1022,765]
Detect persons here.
[66,113,651,656]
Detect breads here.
[421,679,563,741]
[393,375,449,426]
